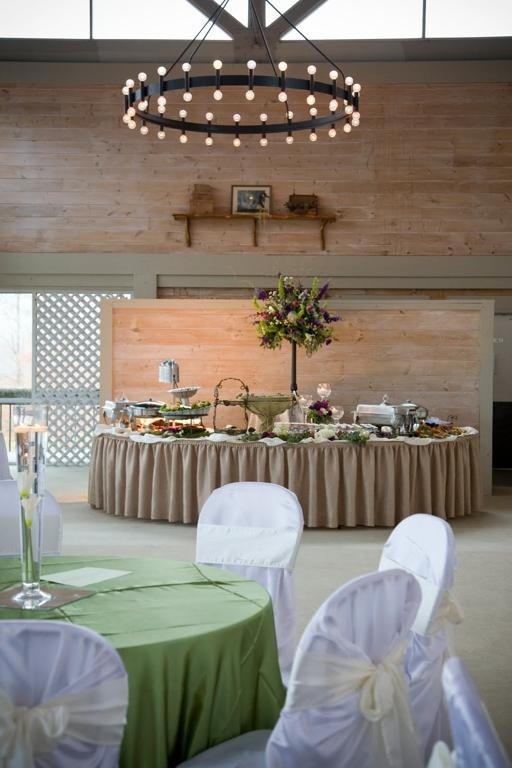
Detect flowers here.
[246,273,340,359]
[308,398,331,417]
[17,471,36,585]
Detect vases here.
[12,460,51,608]
[290,339,297,391]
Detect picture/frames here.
[232,185,271,213]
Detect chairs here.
[377,513,463,768]
[0,479,62,556]
[428,657,512,767]
[1,619,130,768]
[194,481,304,682]
[171,569,429,768]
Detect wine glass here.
[329,405,344,425]
[317,383,331,401]
[297,393,312,423]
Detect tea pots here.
[396,399,428,436]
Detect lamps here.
[123,1,362,147]
